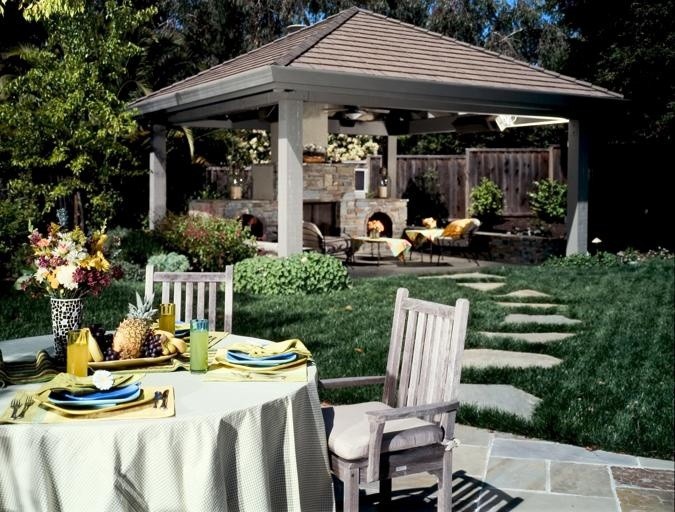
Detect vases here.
[50,298,83,353]
[372,230,382,239]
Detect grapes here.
[143,332,161,357]
[90,324,114,360]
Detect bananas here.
[154,330,187,355]
[76,328,103,362]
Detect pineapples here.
[112,290,157,359]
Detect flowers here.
[372,220,384,232]
[12,219,126,298]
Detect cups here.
[67,330,89,376]
[158,303,175,336]
[190,319,209,374]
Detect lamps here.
[346,105,362,119]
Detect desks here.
[407,229,444,264]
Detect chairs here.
[316,288,470,510]
[303,221,350,265]
[438,222,484,265]
[145,263,233,333]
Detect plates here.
[175,329,190,338]
[65,383,139,400]
[88,352,178,367]
[216,348,307,371]
[228,351,293,360]
[32,389,155,416]
[0,385,174,424]
[225,349,297,366]
